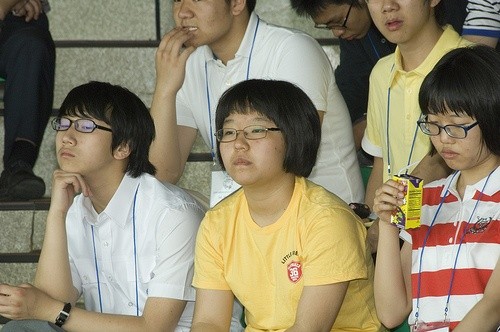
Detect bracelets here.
[54,301,72,328]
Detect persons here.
[373,42,500,332]
[0,81,242,332]
[145,0,363,212]
[290,0,469,170]
[0,0,57,198]
[191,80,389,332]
[464,0,500,48]
[351,0,473,255]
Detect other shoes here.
[0,155,47,200]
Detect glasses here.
[50,117,110,134]
[311,5,352,30]
[417,110,478,140]
[214,123,280,143]
